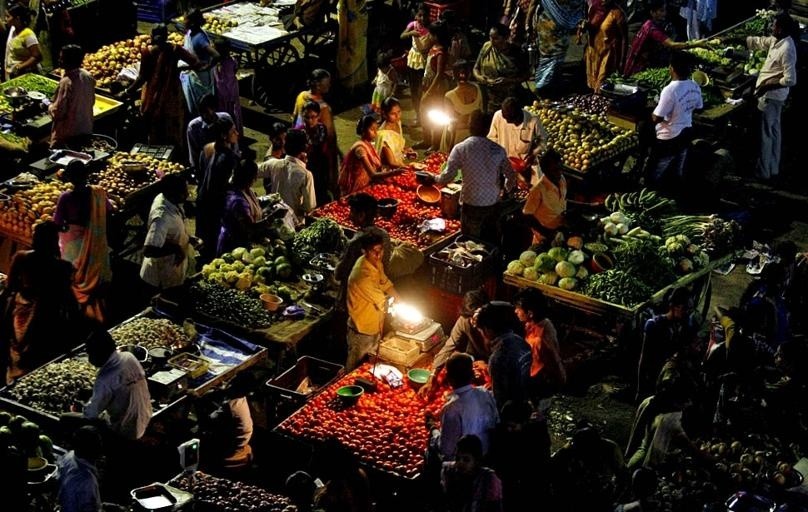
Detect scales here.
[178,438,201,471]
[711,66,742,83]
[147,367,189,397]
[395,321,445,353]
[2,87,48,121]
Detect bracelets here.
[263,216,271,226]
[124,88,132,96]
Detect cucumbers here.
[609,226,661,245]
[604,188,676,224]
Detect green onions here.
[661,214,743,252]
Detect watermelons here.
[0,412,53,457]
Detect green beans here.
[574,267,655,309]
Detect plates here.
[147,347,174,358]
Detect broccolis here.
[657,234,709,276]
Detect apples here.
[82,15,238,88]
[658,437,791,500]
[0,180,75,238]
[524,99,640,172]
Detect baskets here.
[431,234,495,295]
[266,356,345,425]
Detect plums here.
[277,362,492,476]
[312,150,530,248]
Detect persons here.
[369,53,397,110]
[257,67,345,219]
[112,25,195,146]
[194,114,241,247]
[374,96,419,172]
[400,1,718,157]
[197,369,259,457]
[81,329,155,444]
[138,173,197,290]
[281,469,322,512]
[331,193,391,328]
[416,238,808,512]
[314,435,378,511]
[177,4,221,114]
[185,95,237,167]
[332,113,406,194]
[52,158,117,321]
[46,44,96,152]
[419,108,519,248]
[210,34,244,140]
[518,152,570,246]
[209,160,289,255]
[46,423,107,512]
[2,4,45,80]
[485,95,551,173]
[645,52,703,186]
[0,220,79,387]
[342,233,402,372]
[725,12,799,183]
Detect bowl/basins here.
[375,196,400,217]
[338,384,364,406]
[691,71,709,87]
[1,84,26,98]
[770,468,806,491]
[90,132,118,152]
[406,367,432,386]
[593,252,613,272]
[116,344,148,365]
[416,185,442,204]
[4,179,34,192]
[414,168,437,186]
[25,453,46,473]
[119,161,143,170]
[599,81,640,96]
[259,293,285,310]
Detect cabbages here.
[507,247,589,291]
